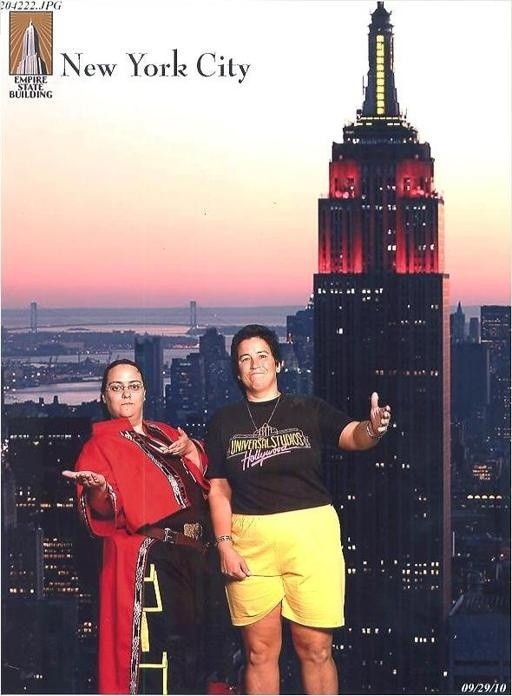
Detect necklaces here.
[245,395,281,441]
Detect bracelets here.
[213,535,233,547]
[367,421,383,441]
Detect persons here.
[62,358,209,694]
[204,324,391,695]
